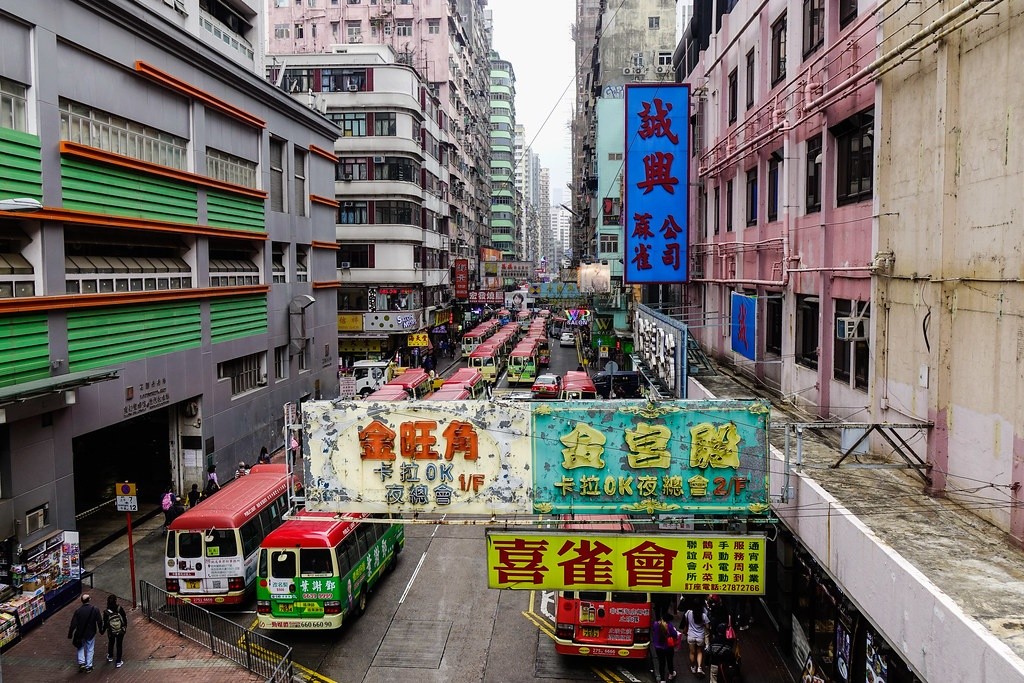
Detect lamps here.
[585,260,592,265]
[619,258,623,264]
[602,259,608,265]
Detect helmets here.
[710,594,719,603]
[716,623,726,634]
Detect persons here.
[67,593,103,673]
[577,325,624,371]
[102,593,127,668]
[652,594,749,683]
[423,310,502,374]
[160,433,298,536]
[513,293,524,308]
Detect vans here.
[549,318,573,339]
[591,369,639,398]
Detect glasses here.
[90,597,91,600]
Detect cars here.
[559,333,575,348]
[530,372,563,399]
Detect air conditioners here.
[429,84,435,90]
[446,289,452,294]
[623,67,645,75]
[349,36,363,42]
[349,85,358,91]
[836,318,868,341]
[415,263,421,269]
[667,65,676,74]
[342,262,350,268]
[374,156,384,163]
[655,65,666,73]
[343,174,352,181]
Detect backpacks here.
[162,493,173,509]
[105,605,128,637]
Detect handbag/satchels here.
[680,597,687,613]
[72,636,82,649]
[666,624,675,647]
[725,615,736,639]
[674,631,683,653]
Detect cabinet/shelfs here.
[21,530,63,592]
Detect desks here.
[0,592,41,640]
[0,616,22,654]
[37,571,95,625]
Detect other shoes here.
[116,661,123,668]
[107,653,114,661]
[705,645,710,651]
[698,668,706,675]
[669,671,676,680]
[80,664,85,671]
[691,666,696,673]
[86,667,93,673]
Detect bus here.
[256,500,405,635]
[162,462,308,607]
[550,589,655,663]
[559,370,599,401]
[350,307,551,402]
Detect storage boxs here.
[23,575,52,594]
